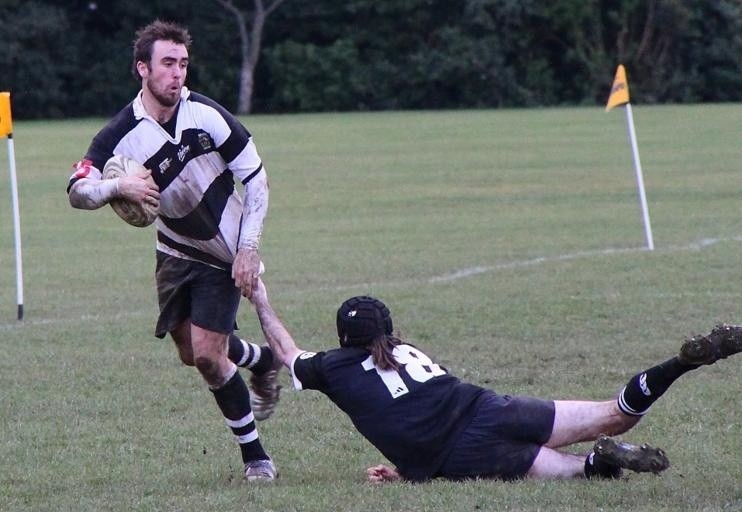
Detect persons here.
[64,22,283,486]
[242,277,740,484]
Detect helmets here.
[336,296,394,344]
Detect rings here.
[144,195,147,200]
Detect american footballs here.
[103,156,160,226]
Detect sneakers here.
[677,322,741,367]
[591,438,670,473]
[246,344,283,422]
[241,457,279,484]
